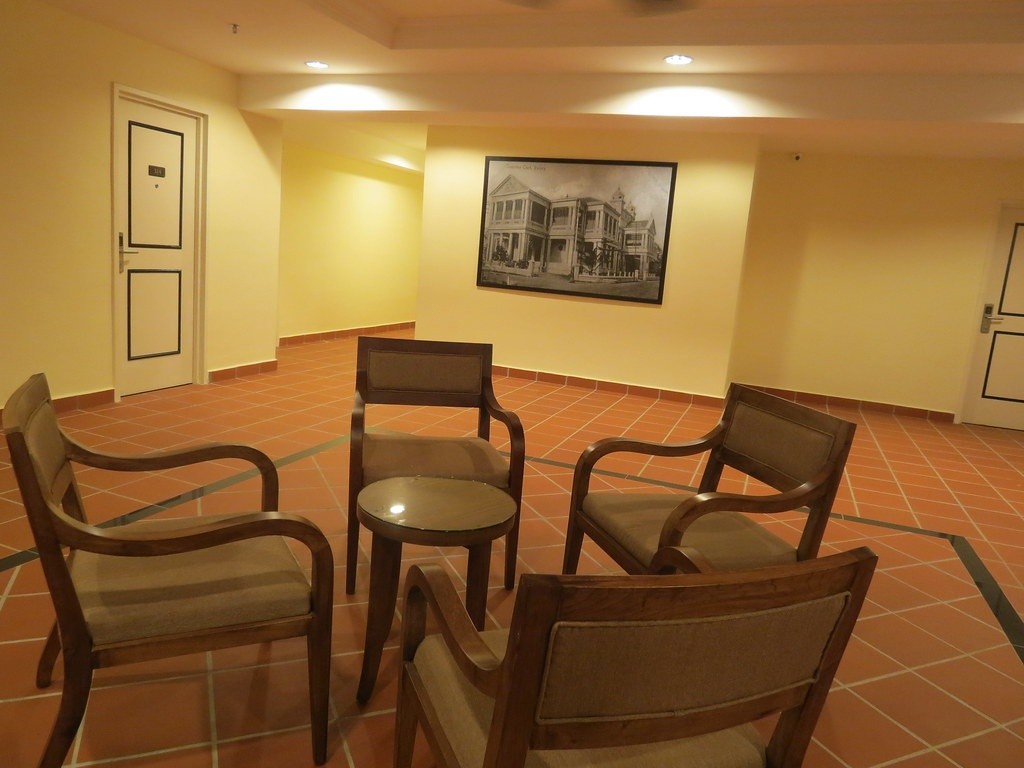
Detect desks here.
[356,478,517,704]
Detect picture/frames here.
[476,155,678,306]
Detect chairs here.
[2,372,337,768]
[563,382,859,576]
[345,333,528,597]
[396,545,880,767]
